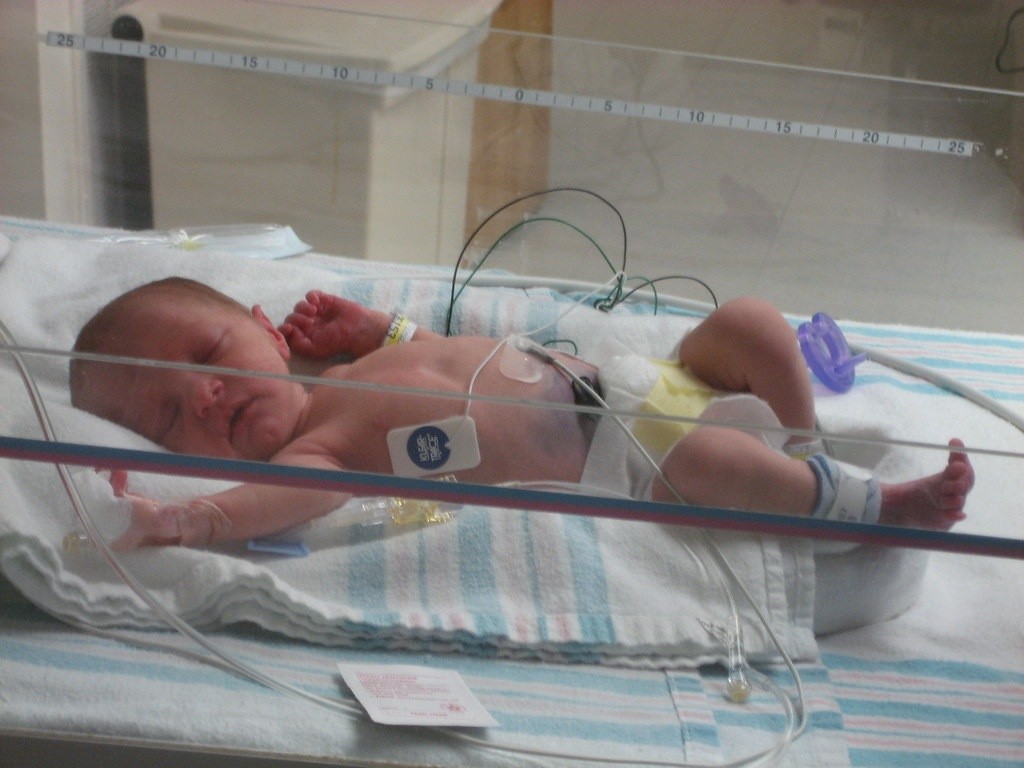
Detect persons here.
[69,277,974,543]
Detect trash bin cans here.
[107,1,506,270]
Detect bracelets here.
[382,312,418,349]
